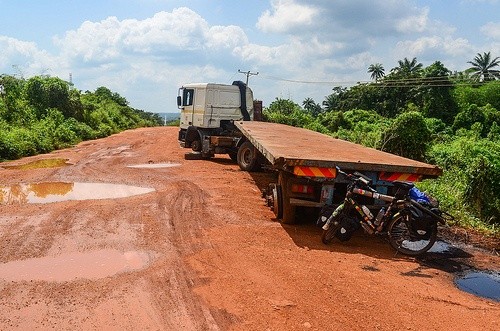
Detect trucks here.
[177,83,441,221]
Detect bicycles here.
[320,164,437,254]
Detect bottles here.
[374,207,385,225]
[360,221,374,235]
[362,205,372,219]
